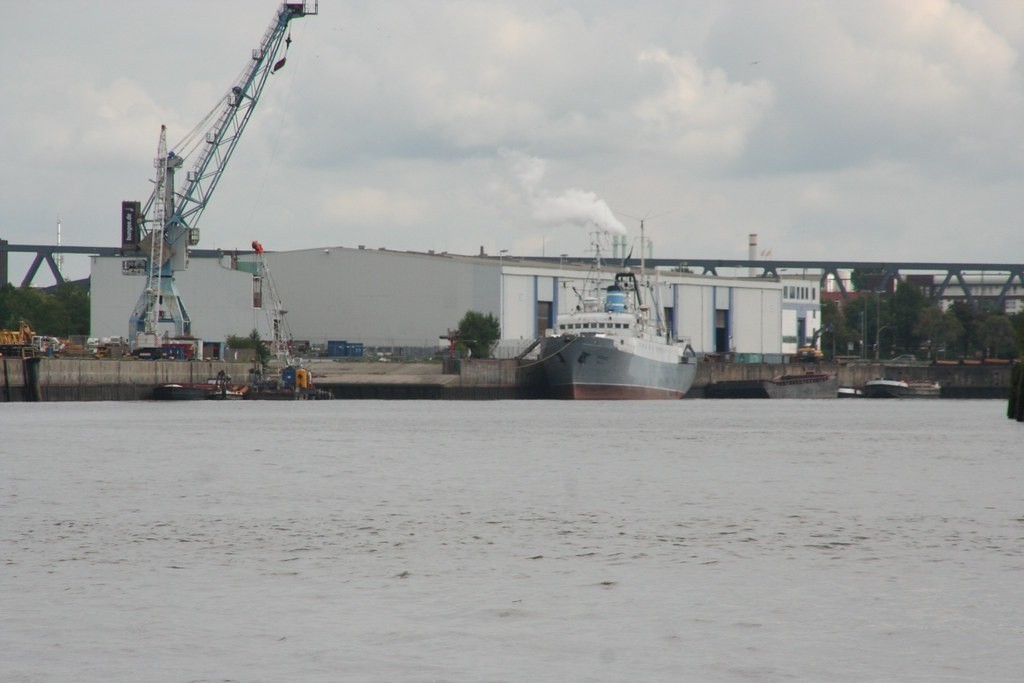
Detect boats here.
[247,241,336,401]
[703,369,840,400]
[151,370,247,400]
[514,219,698,401]
[861,377,941,399]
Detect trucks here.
[59,336,128,360]
[0,336,66,358]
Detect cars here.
[891,354,915,361]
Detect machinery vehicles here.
[797,323,832,364]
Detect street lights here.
[872,291,886,359]
[859,289,872,360]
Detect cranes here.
[128,0,318,361]
